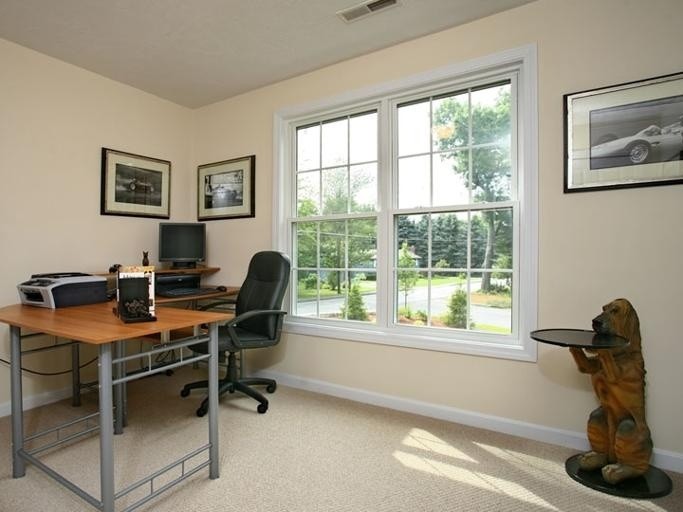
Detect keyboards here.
[159,288,207,297]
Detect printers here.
[16,271,108,310]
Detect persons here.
[205,176,221,209]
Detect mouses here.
[216,286,226,292]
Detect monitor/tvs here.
[158,223,206,269]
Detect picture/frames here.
[561,71,683,196]
[100,146,172,220]
[194,154,255,223]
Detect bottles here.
[142,251,149,266]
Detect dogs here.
[570,297,654,486]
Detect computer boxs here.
[155,273,201,295]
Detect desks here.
[0,283,249,512]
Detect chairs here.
[178,250,291,419]
